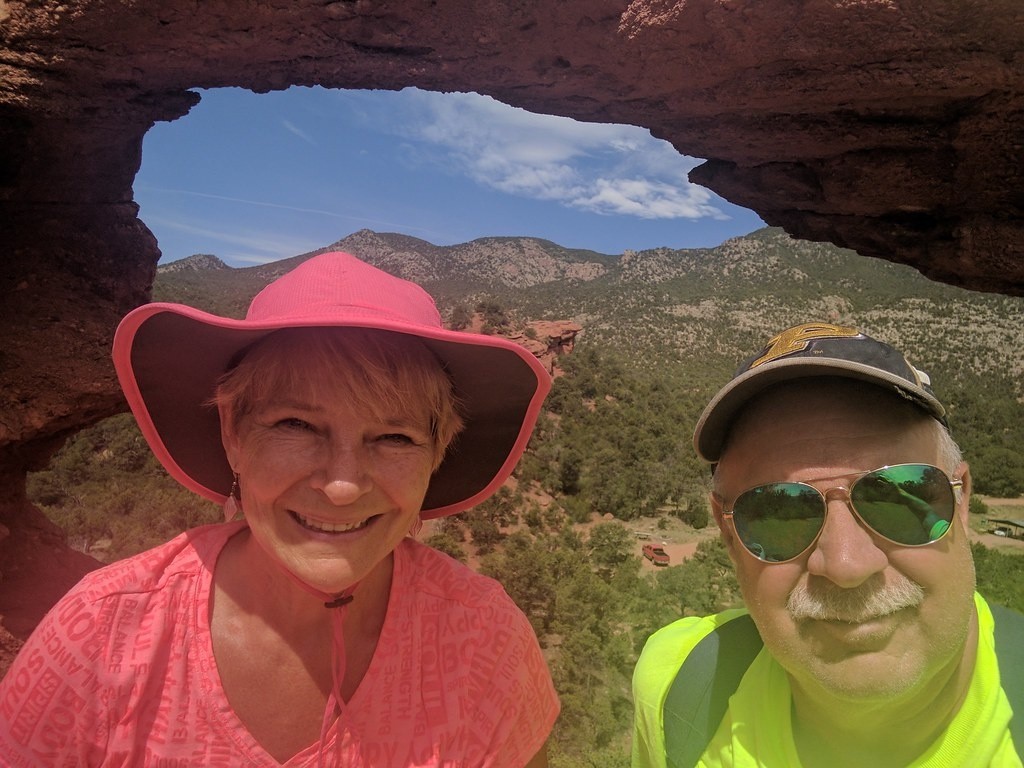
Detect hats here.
[690,323,946,461]
[110,251,551,520]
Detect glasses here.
[720,463,965,566]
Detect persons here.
[0,250,563,767]
[630,320,1022,767]
